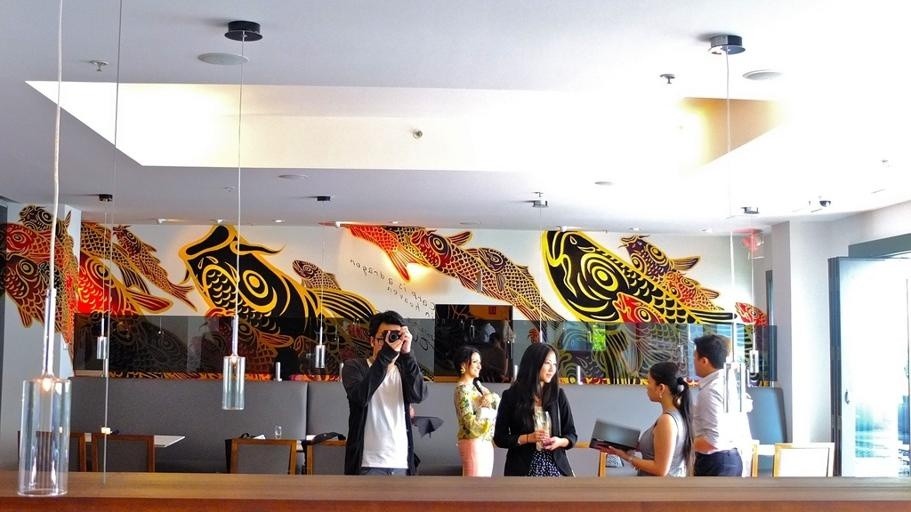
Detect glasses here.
[374,337,385,346]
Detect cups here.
[273,425,282,438]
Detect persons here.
[481,332,510,382]
[339,310,429,475]
[692,335,743,476]
[453,346,501,477]
[598,362,693,478]
[493,343,578,476]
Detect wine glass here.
[537,409,552,449]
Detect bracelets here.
[527,433,529,444]
[628,455,634,462]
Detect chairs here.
[18,430,86,472]
[689,440,759,477]
[565,442,606,476]
[91,432,156,472]
[773,442,835,477]
[230,438,297,474]
[307,440,346,474]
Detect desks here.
[86,433,185,448]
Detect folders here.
[588,420,640,453]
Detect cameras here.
[383,330,400,343]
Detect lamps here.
[315,196,331,369]
[17,0,73,497]
[710,34,746,414]
[750,207,759,372]
[97,195,112,360]
[223,21,263,411]
